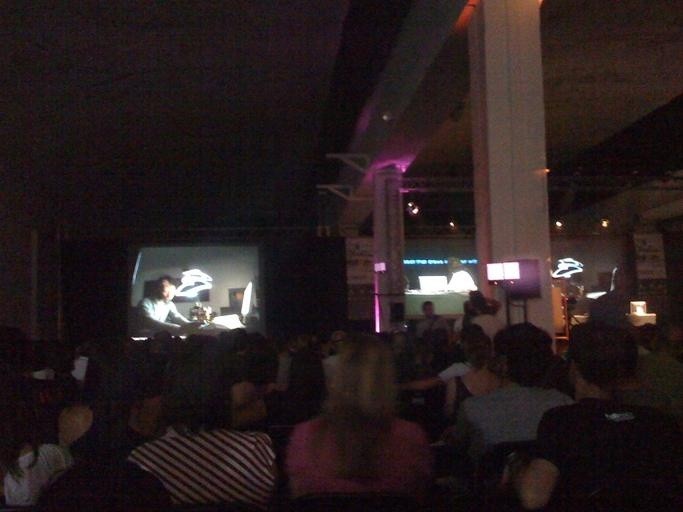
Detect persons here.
[0,323,682,512]
[132,273,206,337]
[415,301,452,336]
[454,291,507,328]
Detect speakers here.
[506,258,541,299]
